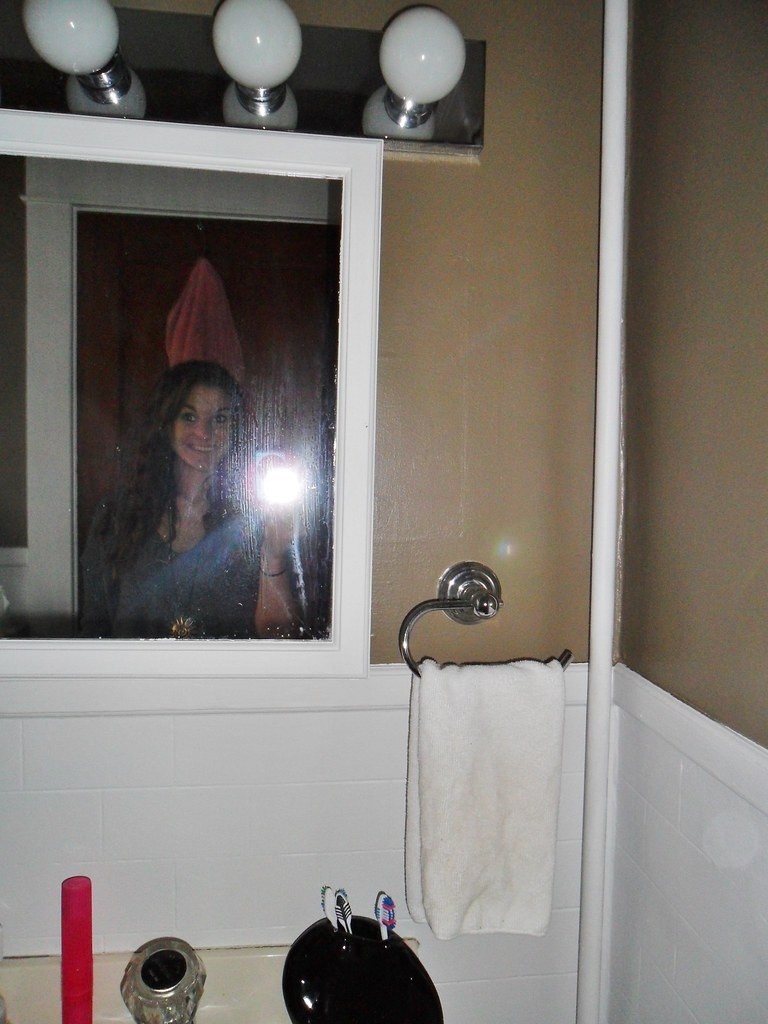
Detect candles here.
[61,876,93,1024]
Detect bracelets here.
[259,566,287,577]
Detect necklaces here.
[164,498,209,639]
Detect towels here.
[163,258,246,384]
[404,659,564,942]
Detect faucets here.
[119,937,207,1023]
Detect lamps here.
[362,7,468,115]
[22,0,123,90]
[66,68,146,119]
[211,1,304,101]
[223,82,299,132]
[362,87,436,141]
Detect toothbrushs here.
[321,884,398,941]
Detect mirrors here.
[0,106,382,678]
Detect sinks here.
[2,941,417,1024]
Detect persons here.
[79,361,303,638]
